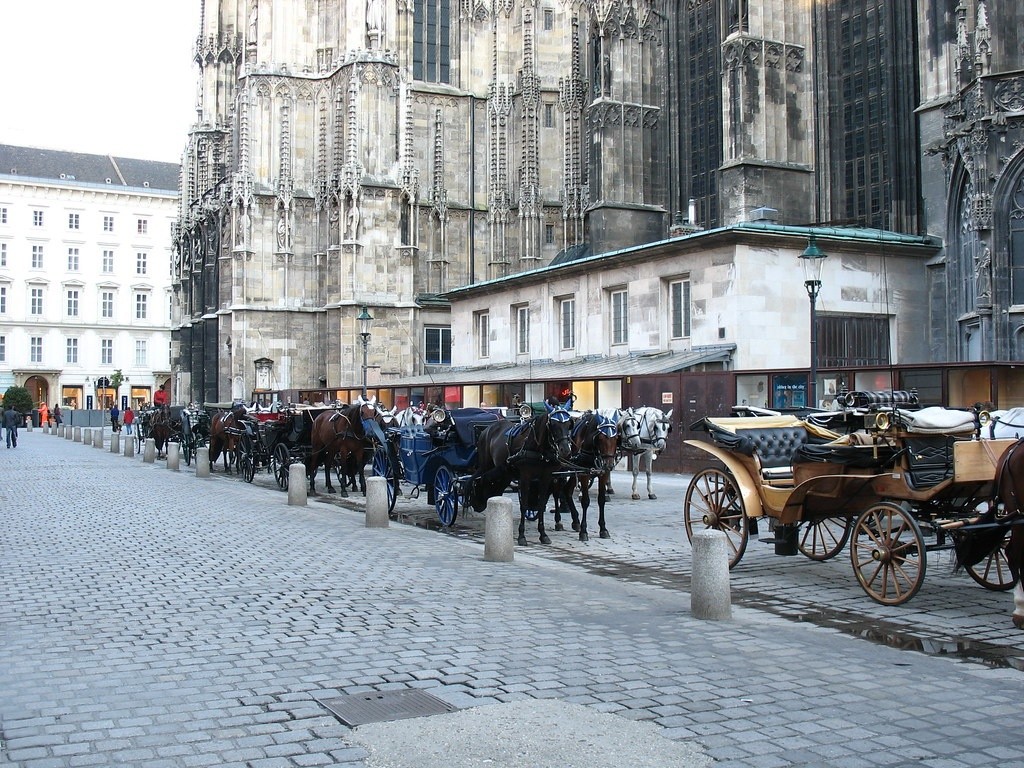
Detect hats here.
[159,385,165,391]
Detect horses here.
[568,406,674,502]
[209,401,274,474]
[310,395,426,496]
[470,394,620,545]
[993,437,1024,631]
[151,404,171,460]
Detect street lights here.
[356,305,375,400]
[797,233,829,407]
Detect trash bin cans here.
[23,413,33,427]
[32,409,39,427]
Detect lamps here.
[144,182,149,188]
[85,377,90,382]
[10,169,16,174]
[60,174,65,179]
[106,178,111,183]
[126,377,130,382]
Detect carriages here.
[682,387,1023,635]
[133,391,676,547]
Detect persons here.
[38,401,62,428]
[0,403,20,449]
[303,398,309,405]
[822,399,833,410]
[154,385,167,407]
[111,405,119,431]
[124,407,134,435]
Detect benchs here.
[703,415,823,472]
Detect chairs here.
[900,426,993,486]
